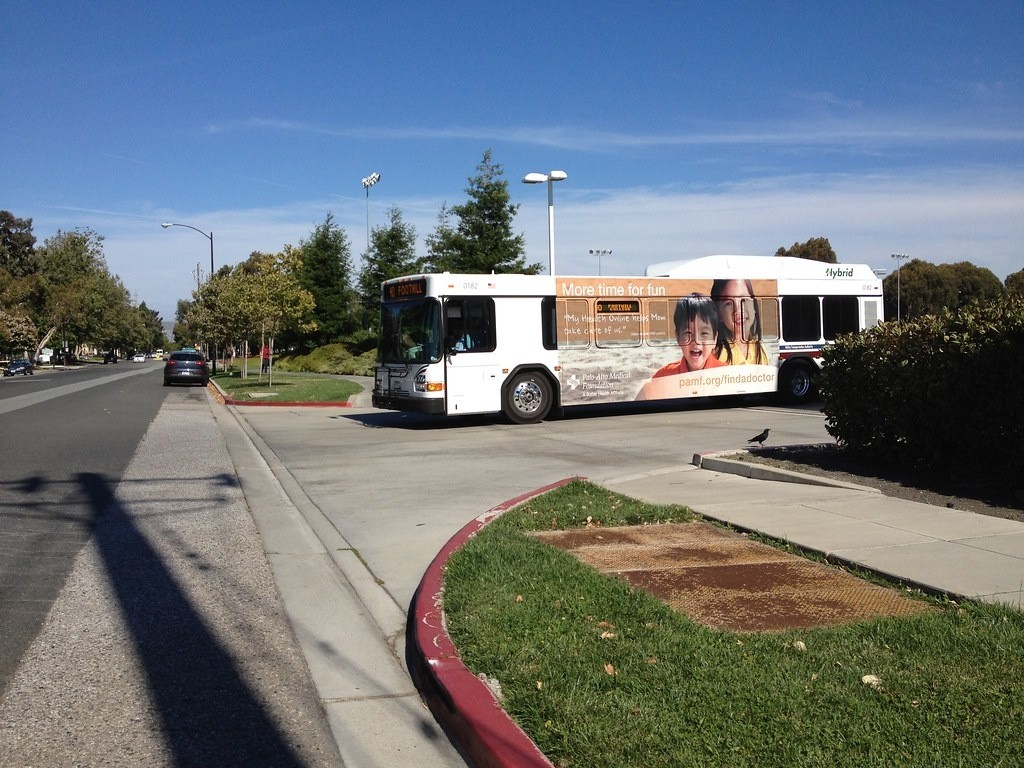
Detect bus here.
[371,264,885,423]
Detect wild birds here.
[748,428,771,446]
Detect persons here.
[259,344,269,374]
[633,291,727,401]
[709,279,770,368]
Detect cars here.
[163,351,210,386]
[133,354,146,363]
[3,359,34,377]
[104,353,118,364]
[149,349,170,360]
[32,348,76,366]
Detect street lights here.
[521,170,568,276]
[191,260,204,299]
[589,249,612,276]
[361,172,381,261]
[160,223,214,276]
[891,252,910,326]
